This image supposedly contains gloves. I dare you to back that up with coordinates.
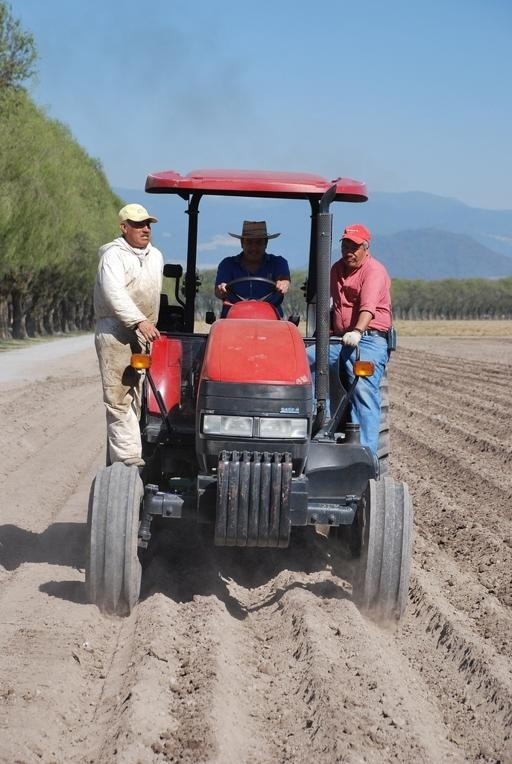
[343,331,362,348]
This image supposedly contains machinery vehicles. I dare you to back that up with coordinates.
[85,166,416,627]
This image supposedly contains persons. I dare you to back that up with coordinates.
[305,224,394,454]
[214,220,291,319]
[91,203,166,466]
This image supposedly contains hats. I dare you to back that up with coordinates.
[118,204,158,225]
[339,225,371,245]
[229,221,281,240]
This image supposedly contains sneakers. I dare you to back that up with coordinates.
[124,457,146,468]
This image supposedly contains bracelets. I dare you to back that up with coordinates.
[353,326,365,335]
